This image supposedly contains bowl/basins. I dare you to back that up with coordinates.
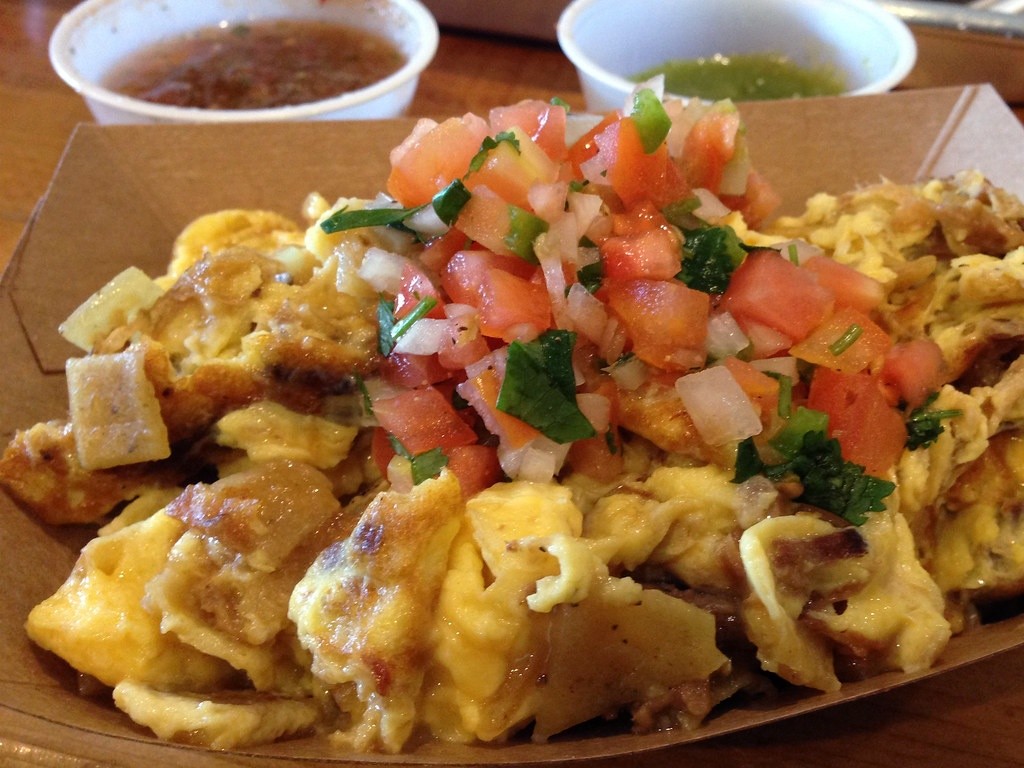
[48,0,442,120]
[556,0,918,118]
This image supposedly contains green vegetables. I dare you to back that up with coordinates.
[318,88,964,530]
